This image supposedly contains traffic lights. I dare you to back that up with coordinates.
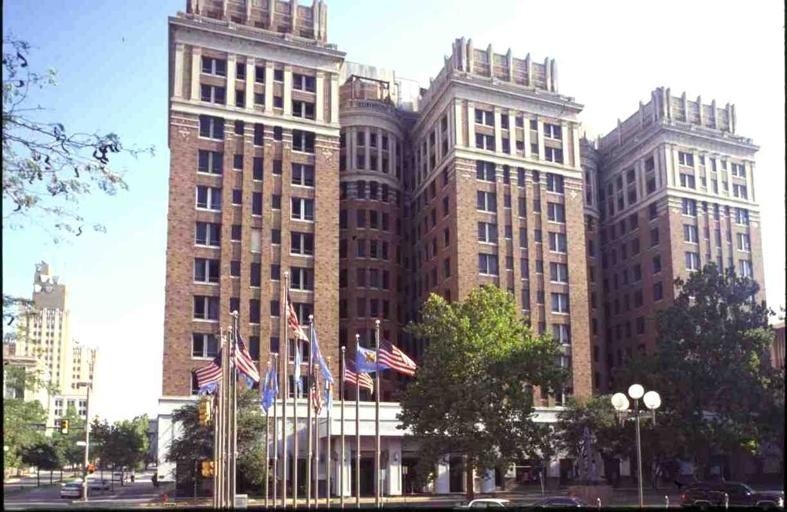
[61,419,68,435]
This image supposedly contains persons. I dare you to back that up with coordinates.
[130,468,135,484]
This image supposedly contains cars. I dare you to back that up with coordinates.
[60,470,131,498]
[454,495,591,509]
[680,481,784,509]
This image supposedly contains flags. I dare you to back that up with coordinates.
[286,289,310,342]
[356,347,390,374]
[343,358,373,394]
[192,328,336,413]
[378,335,417,376]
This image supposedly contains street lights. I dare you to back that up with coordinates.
[579,426,597,498]
[611,384,661,508]
